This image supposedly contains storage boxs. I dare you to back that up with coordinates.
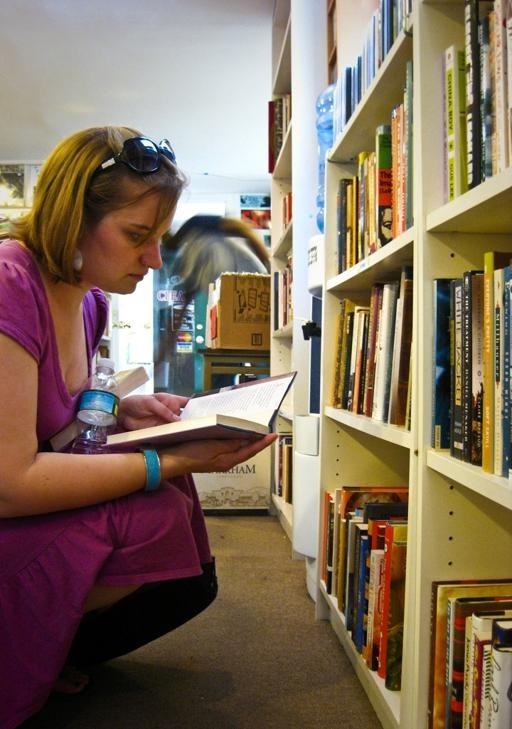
[201,271,271,353]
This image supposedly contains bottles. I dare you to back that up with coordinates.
[70,358,121,455]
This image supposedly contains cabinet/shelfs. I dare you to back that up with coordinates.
[266,0,512,728]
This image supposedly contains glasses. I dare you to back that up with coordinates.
[91,136,177,179]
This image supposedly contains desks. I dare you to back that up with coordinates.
[196,349,270,395]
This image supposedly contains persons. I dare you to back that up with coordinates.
[1,122,282,728]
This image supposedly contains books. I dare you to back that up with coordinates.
[432,252,512,479]
[333,0,413,274]
[428,578,512,729]
[267,93,293,504]
[446,0,512,202]
[101,370,299,437]
[319,265,413,691]
[47,366,150,451]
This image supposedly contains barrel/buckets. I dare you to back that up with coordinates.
[313,82,337,234]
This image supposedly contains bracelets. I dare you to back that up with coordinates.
[139,449,161,492]
